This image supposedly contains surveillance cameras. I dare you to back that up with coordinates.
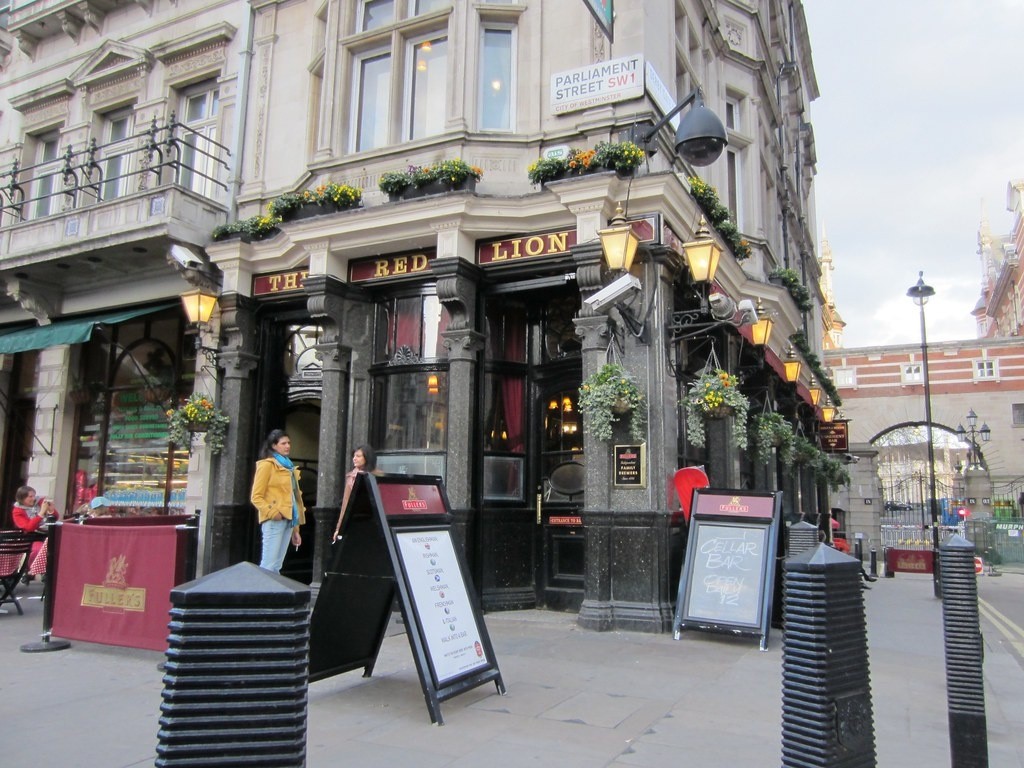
[739,299,758,325]
[583,274,642,315]
[853,456,860,462]
[171,245,203,271]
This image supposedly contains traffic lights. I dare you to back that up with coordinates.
[958,506,966,516]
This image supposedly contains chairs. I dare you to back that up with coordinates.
[1,530,48,614]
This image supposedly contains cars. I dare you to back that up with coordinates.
[884,500,913,511]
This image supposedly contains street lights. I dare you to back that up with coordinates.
[905,269,943,600]
[955,406,992,470]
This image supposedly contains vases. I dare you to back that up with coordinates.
[694,403,734,422]
[388,175,476,202]
[541,165,630,193]
[755,434,782,448]
[769,277,799,304]
[718,229,737,257]
[216,231,261,241]
[794,452,808,464]
[610,401,629,415]
[282,198,352,222]
[186,421,207,433]
[690,189,717,226]
[834,473,845,484]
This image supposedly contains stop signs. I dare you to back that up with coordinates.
[974,558,983,574]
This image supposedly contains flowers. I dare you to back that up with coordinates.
[165,392,231,457]
[577,363,647,443]
[378,157,483,194]
[676,368,751,450]
[527,140,646,190]
[688,176,751,260]
[768,263,813,312]
[748,412,851,492]
[212,180,364,238]
[791,329,842,406]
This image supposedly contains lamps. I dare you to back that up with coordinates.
[417,59,427,71]
[982,497,990,505]
[778,344,802,409]
[549,396,573,413]
[665,214,724,334]
[736,297,775,381]
[595,201,650,347]
[795,372,822,417]
[179,283,225,373]
[968,498,976,505]
[809,395,839,439]
[422,42,432,54]
[864,498,873,505]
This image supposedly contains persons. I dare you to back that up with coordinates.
[14,487,59,585]
[251,430,306,572]
[332,446,382,540]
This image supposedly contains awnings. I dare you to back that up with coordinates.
[0,303,190,453]
[743,344,787,385]
[782,379,826,423]
[688,281,753,345]
